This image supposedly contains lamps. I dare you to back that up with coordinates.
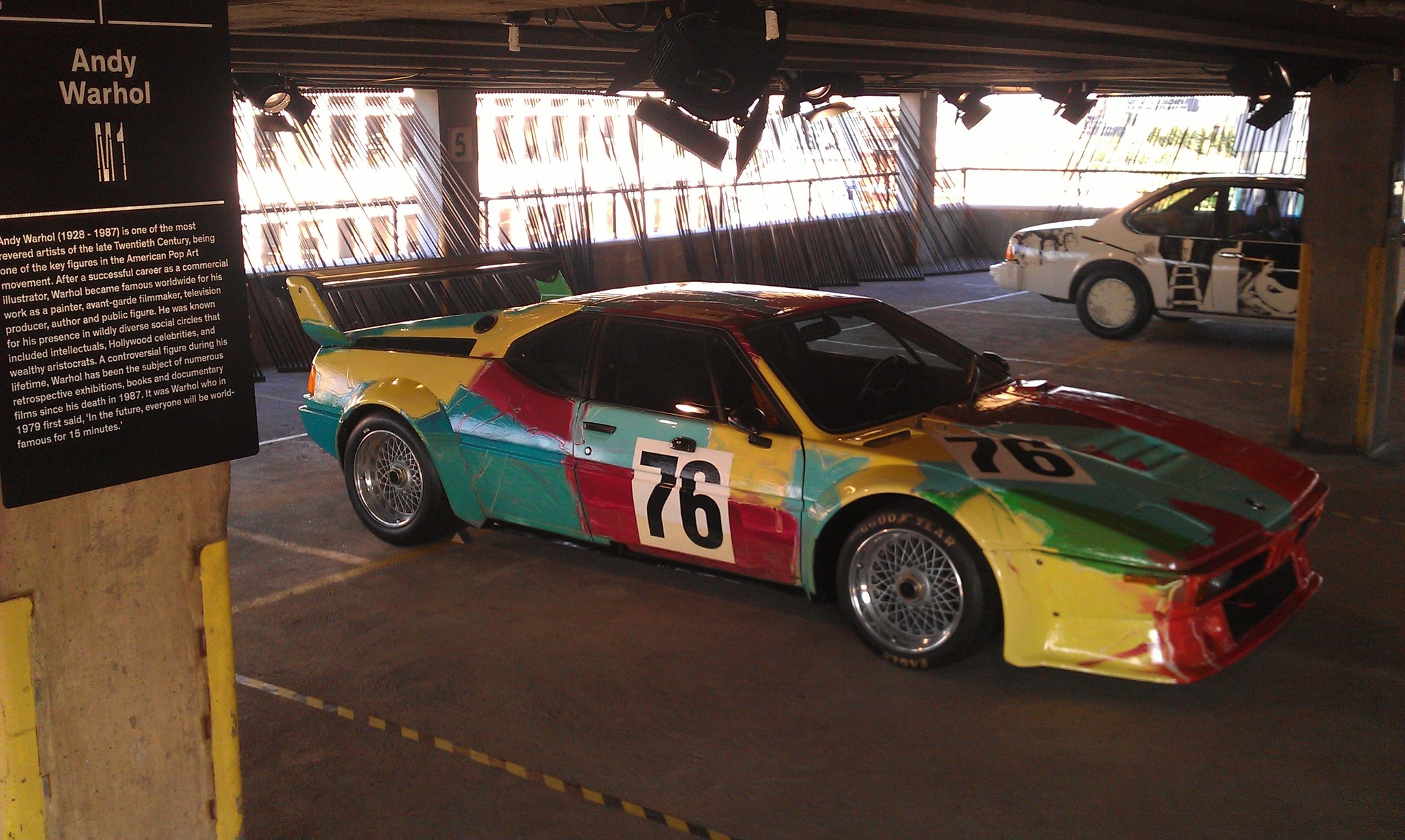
[939,87,994,128]
[1223,56,1351,131]
[606,0,864,178]
[1031,82,1097,124]
[233,72,315,133]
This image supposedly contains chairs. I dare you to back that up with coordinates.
[1160,203,1294,244]
[535,323,833,413]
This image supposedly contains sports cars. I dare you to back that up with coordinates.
[986,175,1405,342]
[284,249,1330,689]
